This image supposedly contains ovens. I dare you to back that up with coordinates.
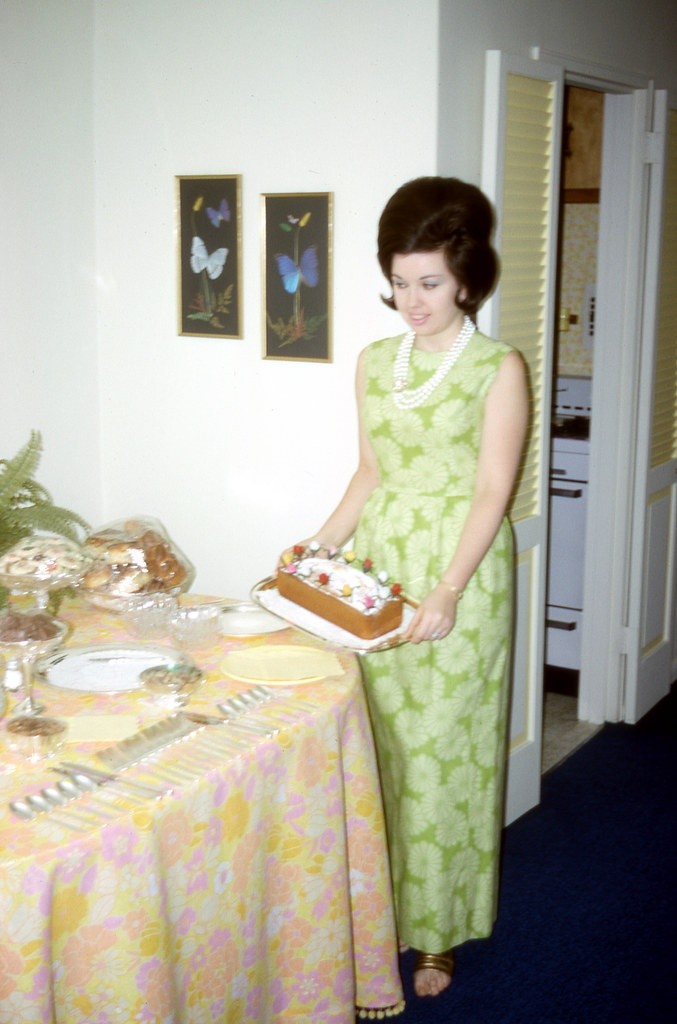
[545,451,588,691]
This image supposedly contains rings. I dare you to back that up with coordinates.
[431,632,440,639]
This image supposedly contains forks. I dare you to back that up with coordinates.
[97,715,258,787]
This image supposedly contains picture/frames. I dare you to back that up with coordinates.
[262,190,337,364]
[172,174,245,340]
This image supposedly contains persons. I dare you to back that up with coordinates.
[273,178,529,996]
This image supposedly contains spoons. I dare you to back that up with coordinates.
[42,790,116,819]
[57,781,131,814]
[237,693,303,721]
[10,801,92,836]
[72,774,147,806]
[248,689,314,716]
[257,686,321,709]
[228,699,294,727]
[26,795,106,829]
[218,704,285,732]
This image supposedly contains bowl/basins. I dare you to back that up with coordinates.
[0,621,68,650]
[5,717,68,752]
[140,663,202,694]
[75,573,195,615]
[120,593,220,652]
[0,568,89,592]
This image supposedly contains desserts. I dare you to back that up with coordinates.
[0,534,88,580]
[276,539,405,640]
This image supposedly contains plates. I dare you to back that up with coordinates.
[215,602,290,636]
[29,643,186,695]
[222,645,326,686]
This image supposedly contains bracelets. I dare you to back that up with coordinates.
[438,581,463,600]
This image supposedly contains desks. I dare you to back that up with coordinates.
[0,591,406,1024]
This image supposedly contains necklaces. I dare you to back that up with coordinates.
[391,315,477,411]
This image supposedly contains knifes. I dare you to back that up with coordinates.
[49,767,161,800]
[60,762,174,796]
[170,710,280,739]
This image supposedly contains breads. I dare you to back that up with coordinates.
[75,518,188,611]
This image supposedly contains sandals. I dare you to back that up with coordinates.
[397,937,410,954]
[413,951,456,1000]
